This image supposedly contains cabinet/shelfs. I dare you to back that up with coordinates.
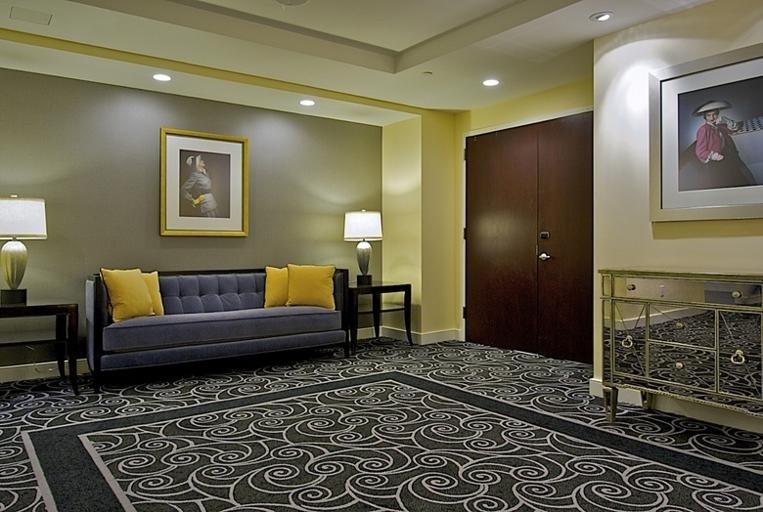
[594,270,762,426]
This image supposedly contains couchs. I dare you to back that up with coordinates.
[81,264,350,391]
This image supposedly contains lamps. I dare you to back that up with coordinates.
[0,192,49,303]
[340,211,384,284]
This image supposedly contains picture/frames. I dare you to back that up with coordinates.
[644,42,762,227]
[157,126,250,238]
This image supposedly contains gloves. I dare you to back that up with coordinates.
[716,116,729,125]
[711,152,723,161]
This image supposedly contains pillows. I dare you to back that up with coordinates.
[283,261,336,311]
[141,271,164,316]
[263,265,290,308]
[100,267,154,322]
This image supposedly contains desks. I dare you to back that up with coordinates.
[0,296,83,399]
[345,281,414,353]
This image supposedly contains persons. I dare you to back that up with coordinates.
[693,100,757,188]
[181,156,220,217]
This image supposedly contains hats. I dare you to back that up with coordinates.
[692,101,732,115]
